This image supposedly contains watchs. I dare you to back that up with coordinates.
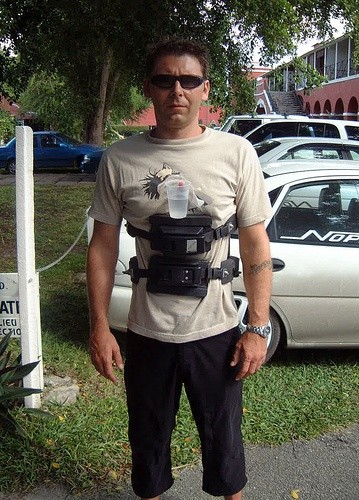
[247,324,270,339]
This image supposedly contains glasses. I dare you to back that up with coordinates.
[150,74,203,89]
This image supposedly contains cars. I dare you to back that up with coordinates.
[0,131,107,175]
[85,158,358,366]
[248,137,358,171]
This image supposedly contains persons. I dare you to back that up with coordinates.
[85,40,273,500]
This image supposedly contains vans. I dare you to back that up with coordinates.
[217,116,313,145]
[238,120,358,152]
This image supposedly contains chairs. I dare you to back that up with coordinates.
[317,187,339,218]
[348,197,359,235]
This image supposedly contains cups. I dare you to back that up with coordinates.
[166,179,191,219]
